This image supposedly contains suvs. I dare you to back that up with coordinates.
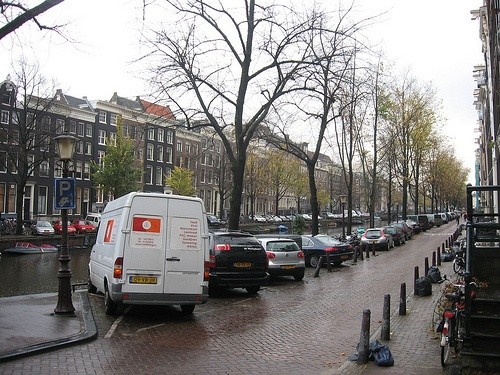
[208,229,305,296]
[207,214,266,226]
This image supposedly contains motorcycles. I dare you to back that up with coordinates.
[333,234,361,258]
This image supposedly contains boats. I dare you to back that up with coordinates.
[3,241,72,253]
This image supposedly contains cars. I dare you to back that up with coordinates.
[31,220,97,237]
[301,234,355,268]
[361,220,422,251]
[261,214,323,223]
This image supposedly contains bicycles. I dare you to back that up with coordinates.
[437,237,480,368]
[0,216,30,236]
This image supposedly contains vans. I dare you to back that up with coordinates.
[85,212,102,232]
[87,191,210,316]
[398,210,461,232]
[322,210,370,219]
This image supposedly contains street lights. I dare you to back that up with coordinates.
[395,200,400,224]
[339,193,348,238]
[419,203,422,215]
